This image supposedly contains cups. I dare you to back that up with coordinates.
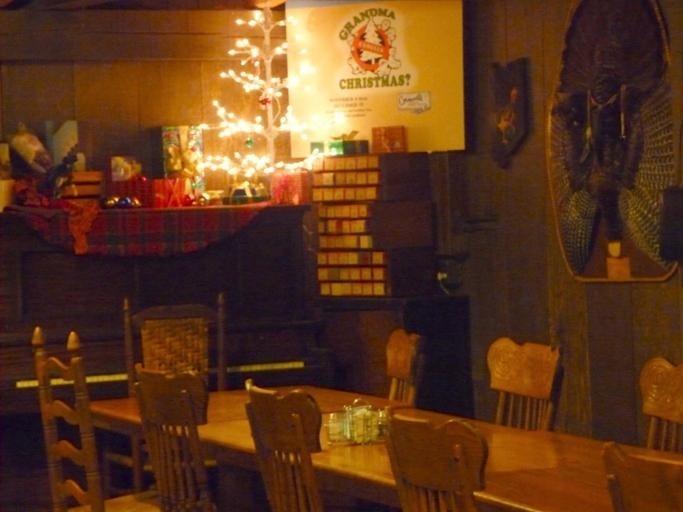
[326,398,388,446]
